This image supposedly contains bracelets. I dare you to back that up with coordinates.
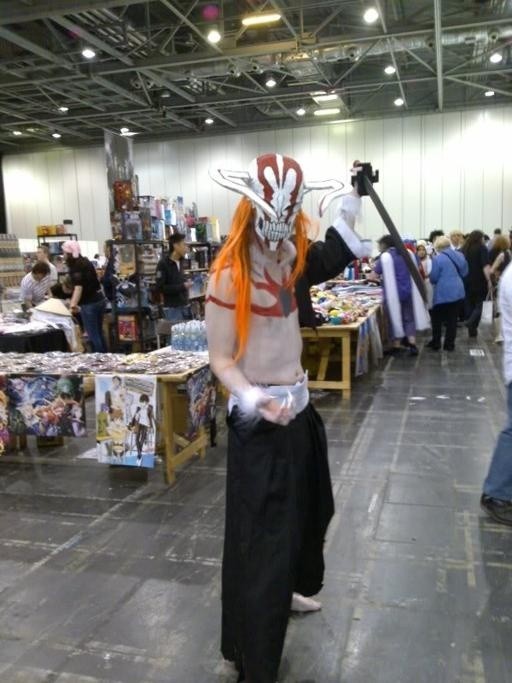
[238,387,269,417]
[341,196,362,216]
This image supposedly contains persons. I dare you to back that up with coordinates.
[480,231,512,526]
[370,228,512,357]
[96,410,131,456]
[203,153,378,683]
[19,239,117,355]
[105,376,127,424]
[155,234,194,345]
[128,395,155,467]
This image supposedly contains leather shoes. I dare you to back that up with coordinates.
[480,493,512,528]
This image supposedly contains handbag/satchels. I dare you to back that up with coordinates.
[481,289,496,325]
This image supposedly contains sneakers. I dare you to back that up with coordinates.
[291,592,321,612]
[387,340,456,358]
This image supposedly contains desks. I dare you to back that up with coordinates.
[298,254,389,399]
[0,227,229,486]
